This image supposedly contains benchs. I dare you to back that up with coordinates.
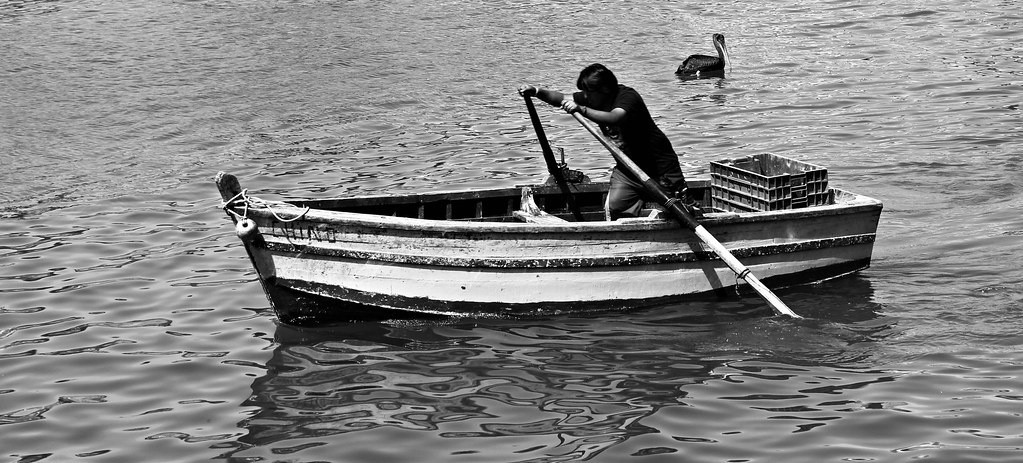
[516,208,569,227]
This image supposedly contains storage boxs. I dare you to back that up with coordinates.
[708,151,830,214]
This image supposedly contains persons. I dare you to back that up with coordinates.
[519,63,694,222]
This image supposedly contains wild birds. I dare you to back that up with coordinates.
[674,33,732,74]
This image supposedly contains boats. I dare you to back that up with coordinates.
[214,171,885,333]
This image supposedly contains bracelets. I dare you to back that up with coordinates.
[533,86,539,96]
[583,105,587,114]
